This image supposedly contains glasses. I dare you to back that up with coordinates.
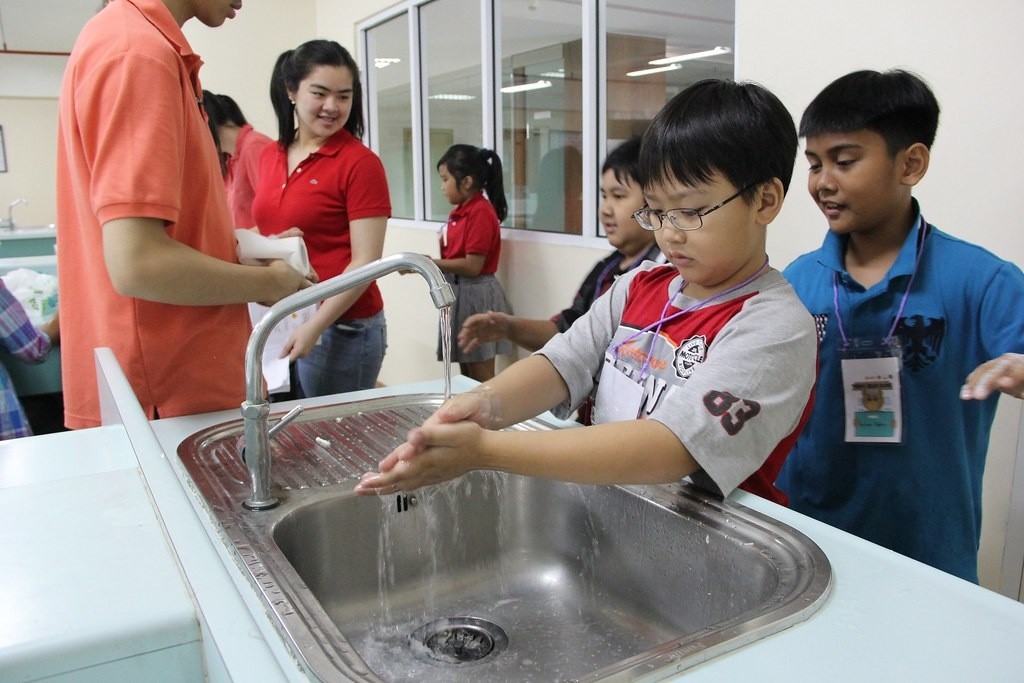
[631,183,752,232]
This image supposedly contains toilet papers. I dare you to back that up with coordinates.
[234,225,310,280]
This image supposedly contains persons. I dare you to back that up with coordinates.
[353,78,821,498]
[458,137,668,354]
[252,39,393,398]
[397,144,512,383]
[58,1,319,429]
[201,89,275,230]
[780,71,1024,588]
[0,278,60,441]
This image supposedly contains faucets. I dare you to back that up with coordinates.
[241,255,455,510]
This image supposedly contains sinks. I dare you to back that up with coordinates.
[176,393,832,682]
